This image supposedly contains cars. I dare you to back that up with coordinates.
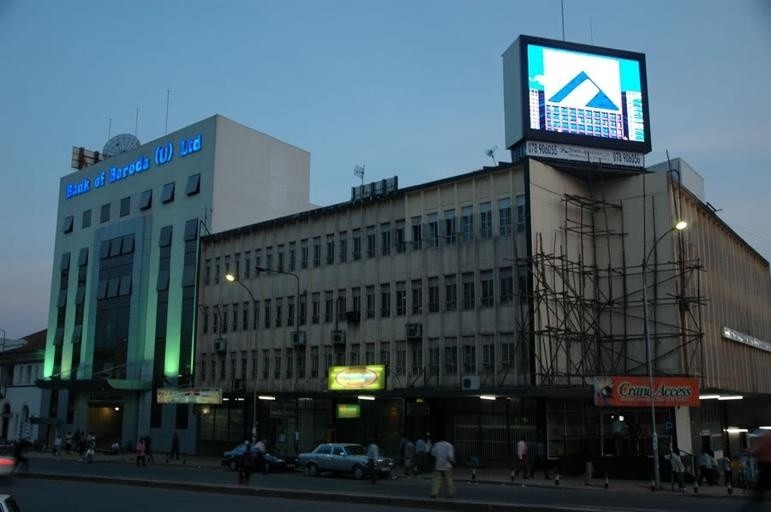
[0,495,20,512]
[221,443,393,480]
[0,455,16,476]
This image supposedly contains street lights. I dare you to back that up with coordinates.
[226,265,300,450]
[643,220,689,491]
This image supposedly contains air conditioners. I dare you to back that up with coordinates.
[291,331,307,346]
[331,331,345,344]
[214,339,226,351]
[406,324,421,338]
[462,375,480,391]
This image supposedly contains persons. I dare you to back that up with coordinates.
[136,436,153,467]
[510,433,553,481]
[168,431,179,461]
[367,438,379,484]
[671,448,750,495]
[401,434,432,477]
[52,429,96,456]
[430,432,456,498]
[232,437,269,485]
[111,439,119,454]
